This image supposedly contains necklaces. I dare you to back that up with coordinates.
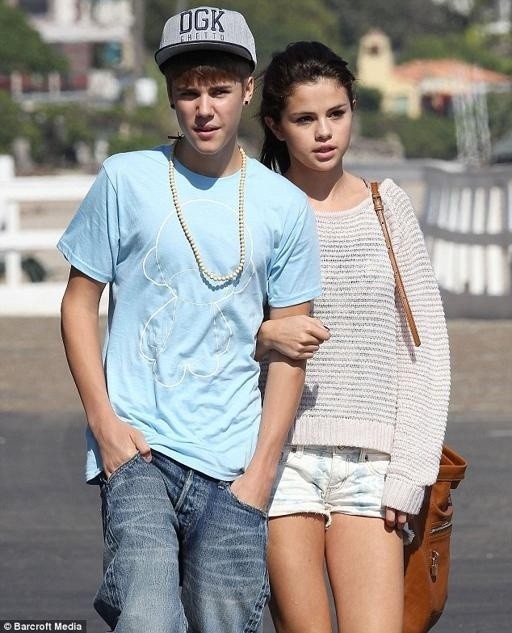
[166,135,248,281]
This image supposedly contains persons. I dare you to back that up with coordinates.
[253,38,453,633]
[55,4,323,633]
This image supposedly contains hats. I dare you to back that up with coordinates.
[153,7,259,78]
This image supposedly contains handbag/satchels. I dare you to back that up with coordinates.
[366,174,468,632]
